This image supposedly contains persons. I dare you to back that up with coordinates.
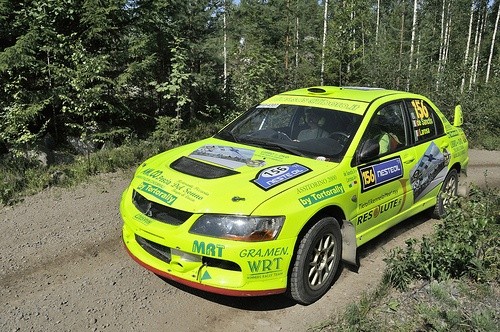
[352,114,391,157]
[296,106,329,142]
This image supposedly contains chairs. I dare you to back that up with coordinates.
[377,114,404,148]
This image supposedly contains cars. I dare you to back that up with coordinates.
[117,84,469,306]
